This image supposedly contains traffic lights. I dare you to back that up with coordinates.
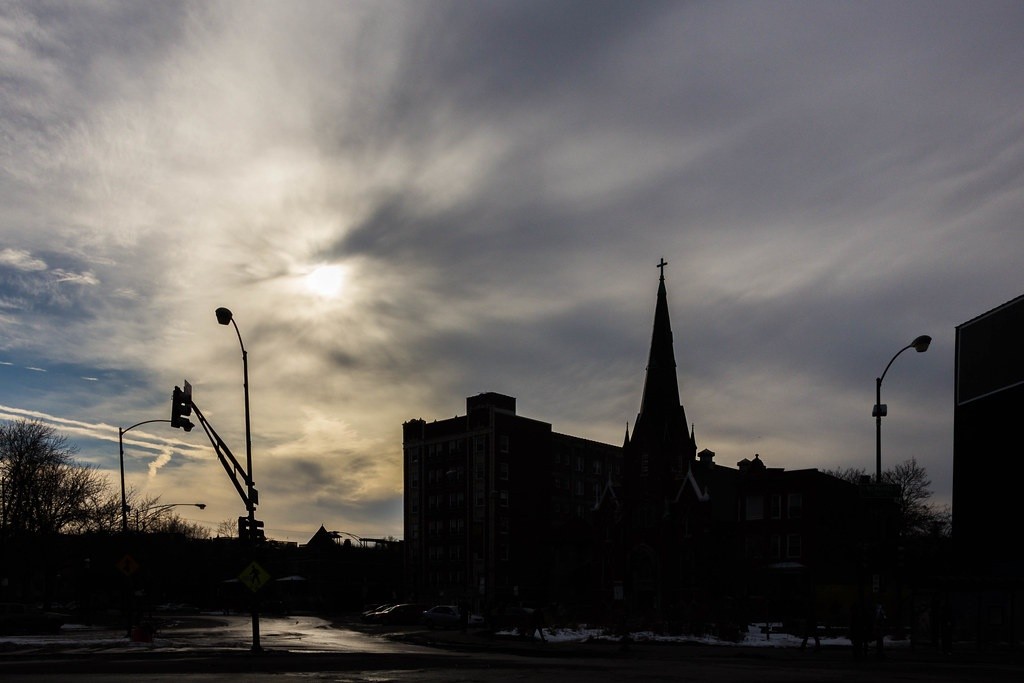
[171,387,194,433]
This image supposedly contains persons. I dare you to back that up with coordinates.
[529,610,546,645]
[800,592,821,651]
[460,607,472,633]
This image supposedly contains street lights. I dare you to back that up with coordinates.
[869,333,932,648]
[136,502,206,530]
[214,306,265,655]
